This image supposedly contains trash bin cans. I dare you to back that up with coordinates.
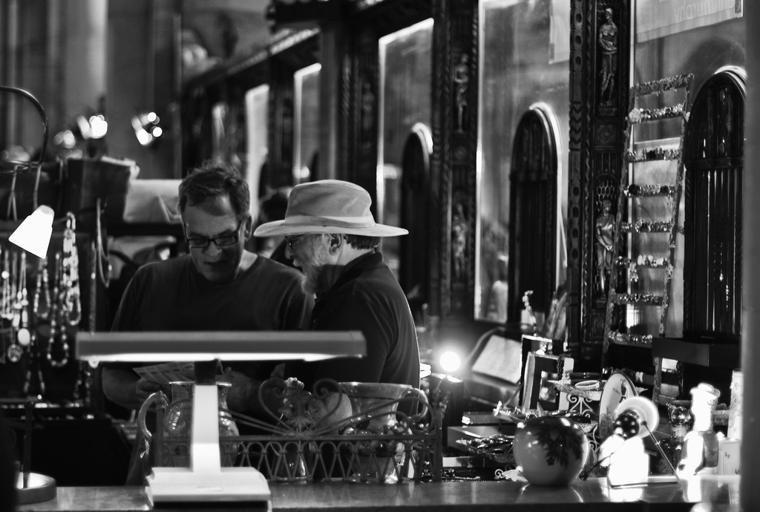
[427,316,441,337]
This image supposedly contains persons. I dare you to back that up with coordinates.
[236,181,420,483]
[102,169,314,482]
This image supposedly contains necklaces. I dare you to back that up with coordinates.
[0,212,82,401]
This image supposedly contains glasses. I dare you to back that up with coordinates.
[284,235,307,250]
[185,221,241,248]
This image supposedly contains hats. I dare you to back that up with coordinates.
[252,178,408,239]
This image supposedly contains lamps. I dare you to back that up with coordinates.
[72,327,374,510]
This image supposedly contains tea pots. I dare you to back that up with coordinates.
[137,381,240,470]
[334,381,428,481]
